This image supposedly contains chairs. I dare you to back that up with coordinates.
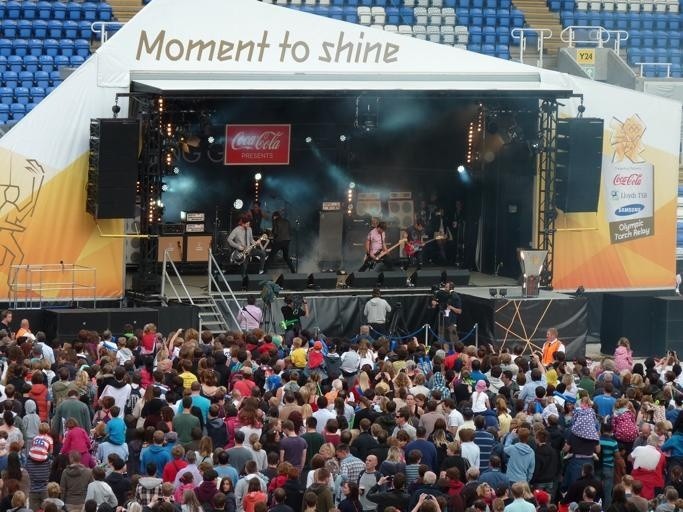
[0,0,123,123]
[261,0,683,78]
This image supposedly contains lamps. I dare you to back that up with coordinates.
[156,95,214,208]
[485,109,545,155]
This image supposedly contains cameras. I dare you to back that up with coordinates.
[157,498,163,501]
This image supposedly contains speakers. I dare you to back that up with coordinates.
[275,273,304,291]
[345,272,378,288]
[441,269,471,286]
[555,116,604,214]
[243,274,274,290]
[379,271,408,287]
[602,294,654,357]
[306,273,337,288]
[409,270,441,286]
[84,119,138,219]
[212,274,242,290]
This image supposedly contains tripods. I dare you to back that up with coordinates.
[387,310,409,335]
[261,303,276,333]
[428,312,453,345]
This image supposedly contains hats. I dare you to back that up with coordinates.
[244,367,252,374]
[314,341,322,349]
[329,344,336,349]
[34,344,43,354]
[475,380,487,392]
[533,490,549,504]
[374,382,390,395]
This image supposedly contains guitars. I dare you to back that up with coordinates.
[405,235,449,256]
[365,238,407,270]
[230,233,268,266]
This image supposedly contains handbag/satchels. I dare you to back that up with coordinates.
[55,453,70,464]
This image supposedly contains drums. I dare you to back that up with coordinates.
[265,227,273,240]
[219,230,232,250]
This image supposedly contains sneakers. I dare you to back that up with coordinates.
[594,453,599,461]
[563,453,573,459]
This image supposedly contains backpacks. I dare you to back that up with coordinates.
[126,386,142,415]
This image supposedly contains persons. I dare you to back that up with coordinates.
[363,288,392,338]
[280,296,309,346]
[225,196,467,277]
[0,308,682,511]
[431,281,463,342]
[237,294,263,331]
